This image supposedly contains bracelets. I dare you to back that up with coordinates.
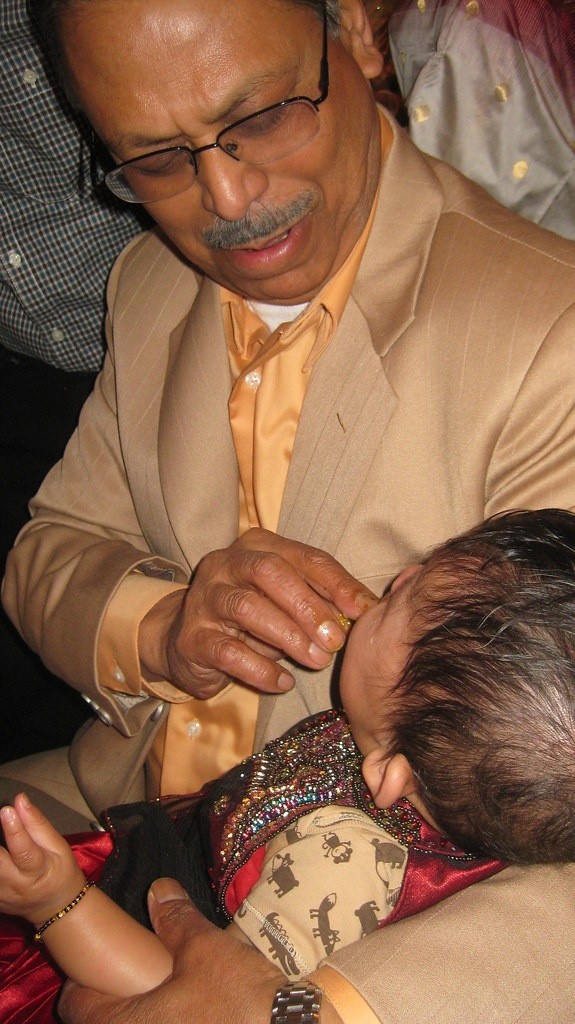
[270,980,324,1024]
[33,882,95,940]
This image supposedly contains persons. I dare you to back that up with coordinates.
[0,509,575,1024]
[0,1,574,1024]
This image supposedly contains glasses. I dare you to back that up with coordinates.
[92,0,329,205]
